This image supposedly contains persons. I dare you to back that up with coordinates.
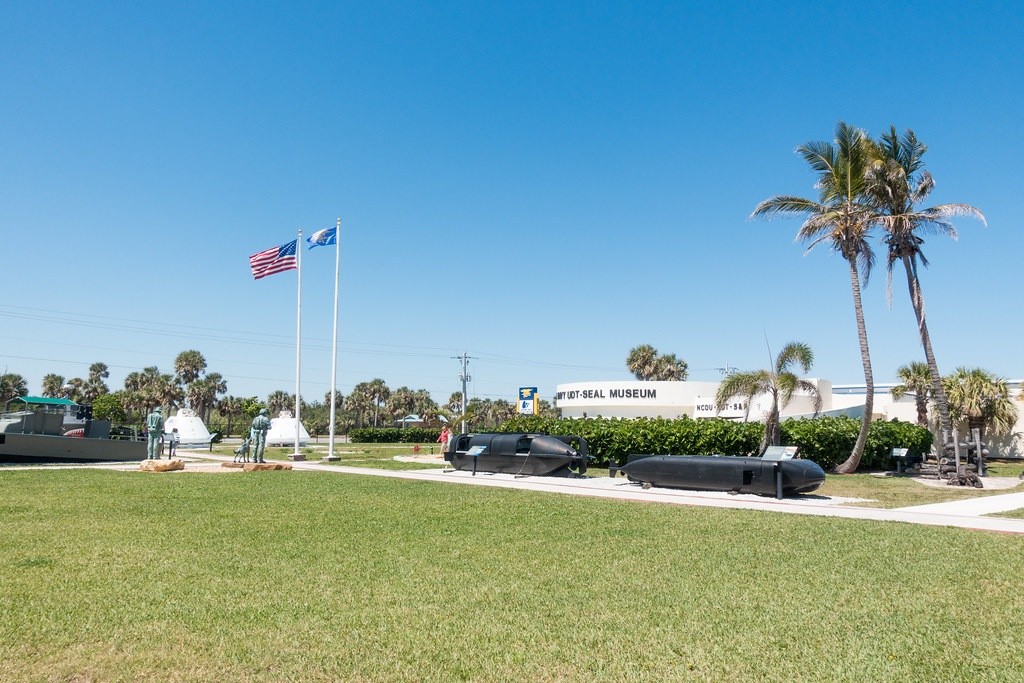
[140,424,148,441]
[146,407,163,459]
[252,409,271,463]
[437,427,448,458]
[171,428,180,456]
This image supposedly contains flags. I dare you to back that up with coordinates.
[248,239,298,280]
[307,226,337,251]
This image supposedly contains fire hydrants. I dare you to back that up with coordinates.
[430,446,433,455]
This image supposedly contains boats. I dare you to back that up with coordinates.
[0,396,164,460]
